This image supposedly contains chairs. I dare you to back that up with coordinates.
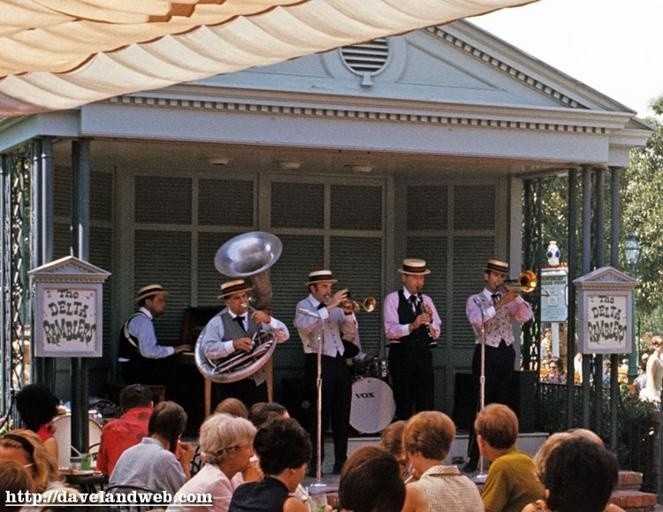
[103,484,169,512]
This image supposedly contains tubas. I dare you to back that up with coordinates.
[195,232,281,383]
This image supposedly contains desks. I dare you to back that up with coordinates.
[172,356,273,419]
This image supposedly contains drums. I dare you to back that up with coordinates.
[364,359,389,378]
[349,377,396,435]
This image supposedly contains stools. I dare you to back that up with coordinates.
[107,382,166,405]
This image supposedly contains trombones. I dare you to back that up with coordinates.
[496,271,550,297]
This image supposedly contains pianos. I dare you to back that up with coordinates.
[176,348,198,367]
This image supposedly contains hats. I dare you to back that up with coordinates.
[134,285,169,305]
[482,259,511,276]
[397,258,431,276]
[304,270,339,288]
[217,279,253,299]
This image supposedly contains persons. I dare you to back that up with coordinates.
[0,384,625,511]
[10,341,33,428]
[462,258,534,473]
[294,270,362,478]
[382,257,443,420]
[194,280,291,410]
[518,321,663,411]
[119,284,199,438]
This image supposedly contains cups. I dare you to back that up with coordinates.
[81,457,91,470]
[401,463,416,483]
[309,483,327,512]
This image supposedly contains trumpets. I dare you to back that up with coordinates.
[327,289,378,316]
